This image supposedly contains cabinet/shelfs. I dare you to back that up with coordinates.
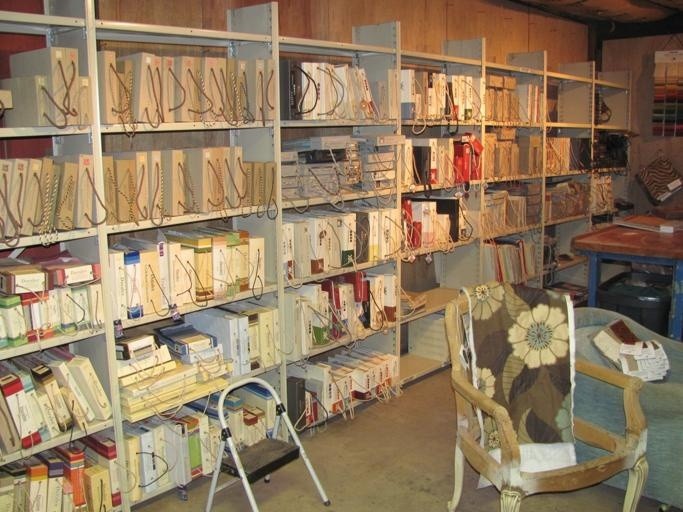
[0,1,640,511]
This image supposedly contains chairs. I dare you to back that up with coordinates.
[573,307,683,511]
[444,284,649,511]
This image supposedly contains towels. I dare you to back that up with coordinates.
[459,286,577,489]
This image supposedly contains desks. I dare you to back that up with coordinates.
[570,215,682,340]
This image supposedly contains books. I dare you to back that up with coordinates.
[483,233,536,287]
[546,280,588,299]
[614,212,672,234]
[398,288,426,321]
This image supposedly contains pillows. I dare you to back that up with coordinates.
[575,317,641,373]
